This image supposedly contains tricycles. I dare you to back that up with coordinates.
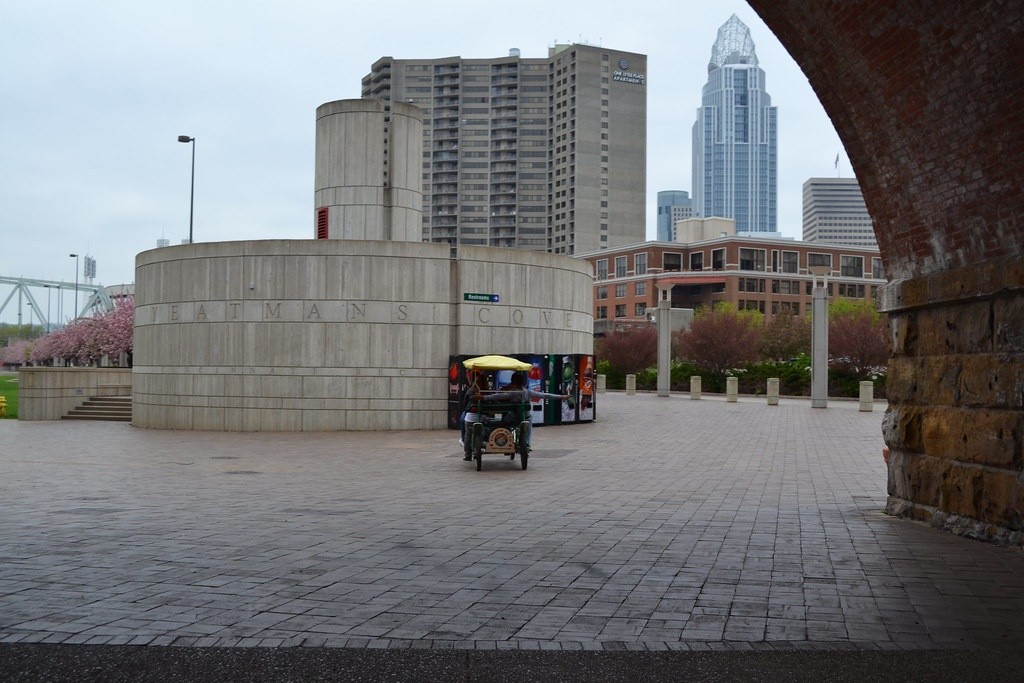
[459,355,533,472]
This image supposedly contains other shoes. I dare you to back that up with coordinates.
[459,438,465,447]
[527,446,533,451]
[463,457,472,461]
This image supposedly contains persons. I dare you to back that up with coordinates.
[459,371,571,461]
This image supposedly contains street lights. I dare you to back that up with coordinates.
[26,302,33,339]
[55,285,60,329]
[18,314,23,327]
[44,285,51,334]
[69,254,79,326]
[177,135,195,244]
